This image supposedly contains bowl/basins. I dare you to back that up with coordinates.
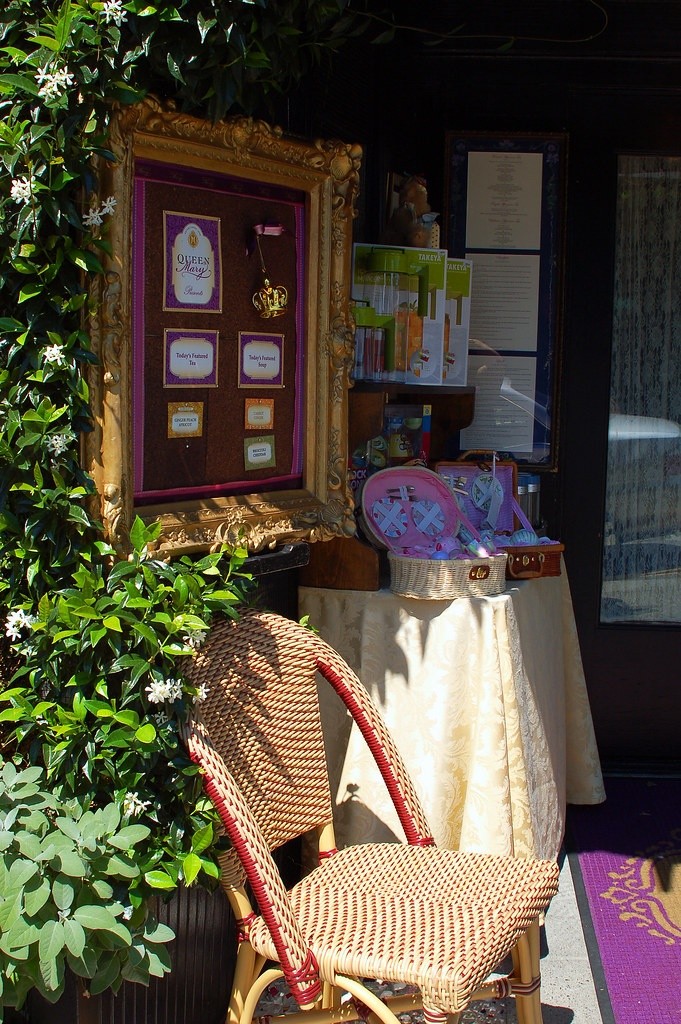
[384,416,404,429]
[404,417,422,430]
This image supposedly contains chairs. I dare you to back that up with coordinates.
[177,607,561,1024]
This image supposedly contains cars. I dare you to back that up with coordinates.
[495,377,680,568]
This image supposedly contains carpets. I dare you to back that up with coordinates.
[562,776,681,1024]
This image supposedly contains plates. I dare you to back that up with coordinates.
[371,434,390,450]
[370,451,387,468]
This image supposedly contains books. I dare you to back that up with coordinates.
[350,242,472,387]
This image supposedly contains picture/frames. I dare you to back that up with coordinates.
[75,90,359,586]
[441,125,572,476]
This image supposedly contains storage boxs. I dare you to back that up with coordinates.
[351,242,448,387]
[443,257,474,387]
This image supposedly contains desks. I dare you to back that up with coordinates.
[297,552,606,927]
